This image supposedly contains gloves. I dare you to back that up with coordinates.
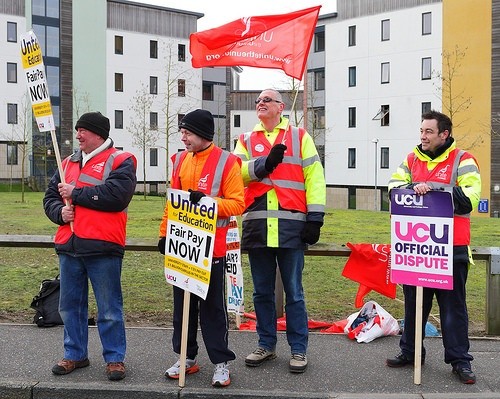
[158,238,166,256]
[188,188,206,205]
[268,144,287,164]
[301,220,322,244]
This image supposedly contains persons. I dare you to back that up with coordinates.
[387,112,479,385]
[158,110,245,387]
[233,86,329,373]
[43,111,137,381]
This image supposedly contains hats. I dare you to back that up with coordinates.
[75,112,110,140]
[179,109,214,141]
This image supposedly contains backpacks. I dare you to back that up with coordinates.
[30,274,64,328]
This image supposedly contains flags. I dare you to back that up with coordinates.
[187,4,322,82]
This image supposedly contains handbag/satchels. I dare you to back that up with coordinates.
[344,301,399,343]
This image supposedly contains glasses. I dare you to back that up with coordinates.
[255,96,282,103]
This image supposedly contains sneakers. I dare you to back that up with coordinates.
[452,365,476,384]
[52,355,89,375]
[245,344,276,367]
[106,361,126,379]
[165,356,199,379]
[289,352,307,373]
[386,353,425,367]
[211,363,230,387]
